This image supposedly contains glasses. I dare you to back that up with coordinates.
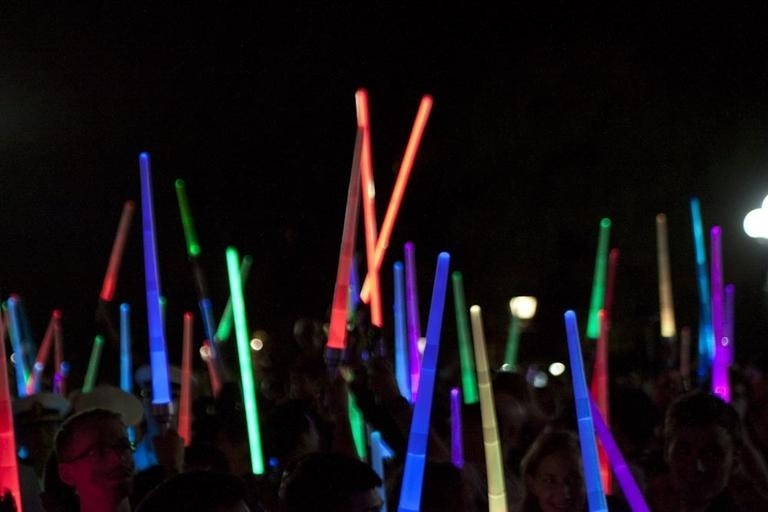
[66,437,135,462]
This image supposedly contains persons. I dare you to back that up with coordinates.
[4,311,767,512]
